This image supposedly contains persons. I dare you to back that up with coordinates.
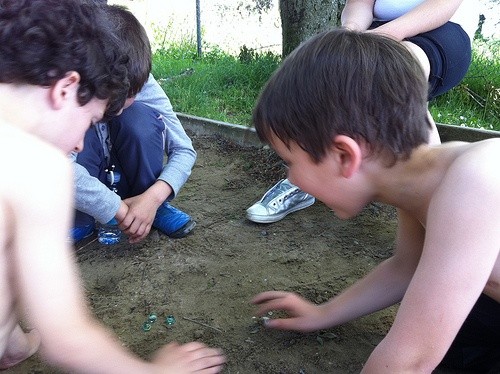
[245,0,481,224]
[251,28,500,374]
[0,0,228,374]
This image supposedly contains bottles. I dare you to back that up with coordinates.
[98,173,123,246]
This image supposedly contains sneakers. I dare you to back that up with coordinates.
[245,178,315,223]
[71,220,97,243]
[152,203,196,238]
